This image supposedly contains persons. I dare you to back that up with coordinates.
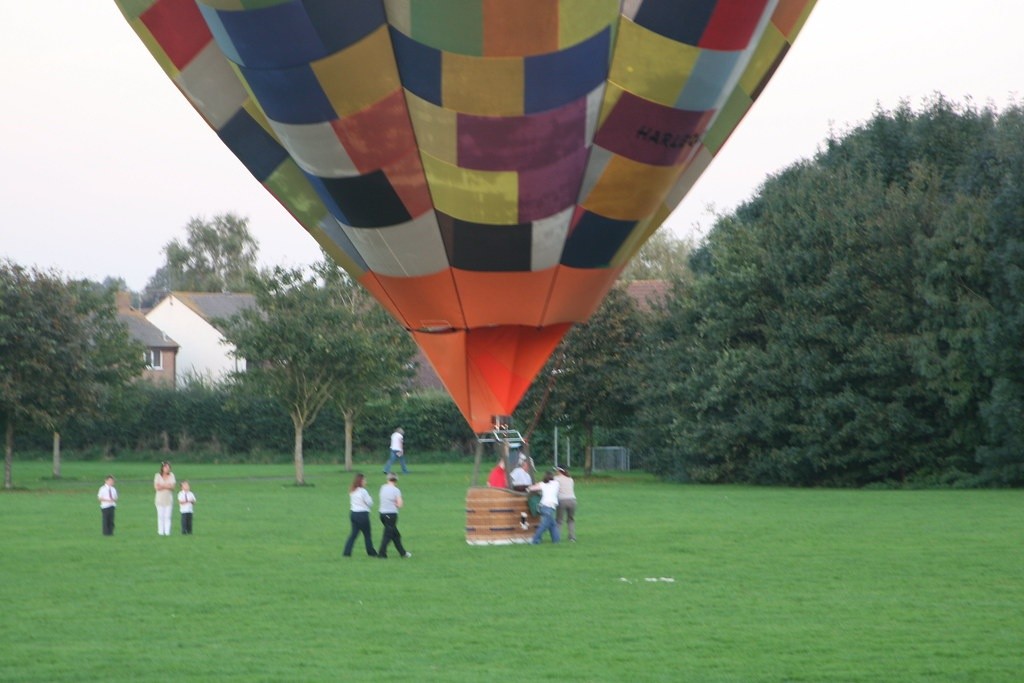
[486,457,511,489]
[382,427,410,475]
[525,472,561,544]
[551,463,576,543]
[177,480,196,535]
[343,473,378,557]
[377,472,412,558]
[97,475,119,536]
[508,460,533,530]
[154,461,177,535]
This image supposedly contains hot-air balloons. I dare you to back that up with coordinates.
[115,0,821,544]
